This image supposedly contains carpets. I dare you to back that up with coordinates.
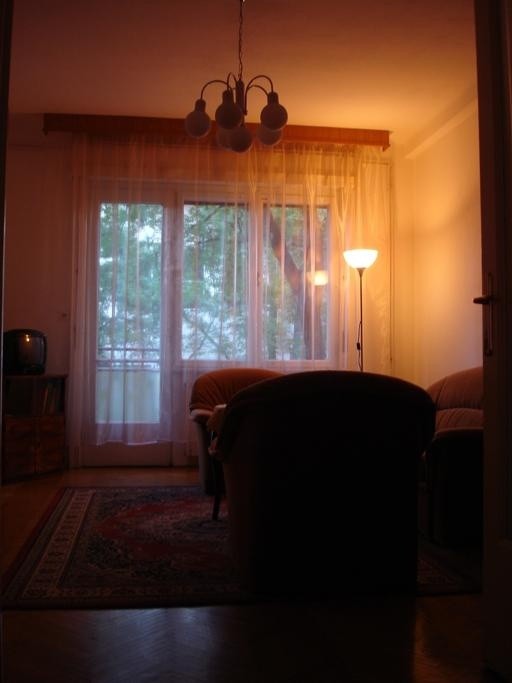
[4,485,485,608]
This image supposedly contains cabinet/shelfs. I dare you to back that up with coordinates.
[3,374,69,480]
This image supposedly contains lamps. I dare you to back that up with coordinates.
[184,0,290,154]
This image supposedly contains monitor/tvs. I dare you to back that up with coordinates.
[3,328,47,375]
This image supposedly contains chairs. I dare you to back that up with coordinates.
[205,371,436,602]
[191,369,294,522]
[416,366,482,557]
